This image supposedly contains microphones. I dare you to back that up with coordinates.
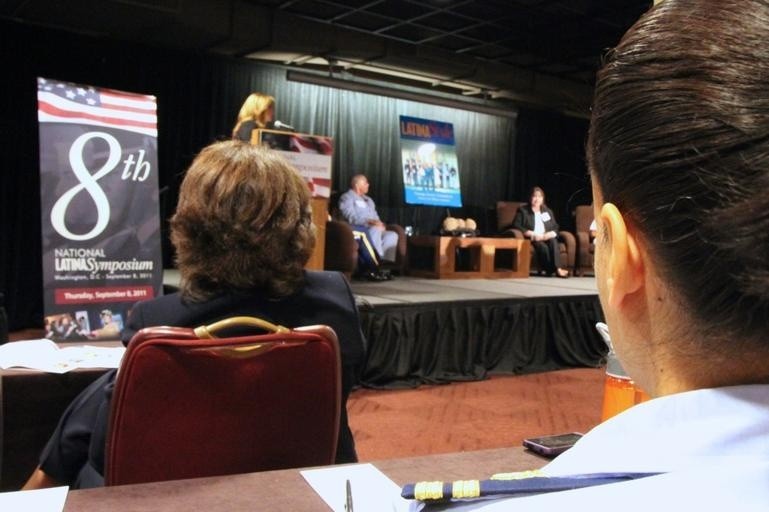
[275,120,296,131]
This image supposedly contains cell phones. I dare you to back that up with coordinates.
[521,431,586,457]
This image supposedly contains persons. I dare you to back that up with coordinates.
[230,93,277,141]
[511,186,570,278]
[9,137,373,488]
[391,0,769,512]
[98,308,114,327]
[338,173,400,282]
[400,158,455,196]
[42,311,87,341]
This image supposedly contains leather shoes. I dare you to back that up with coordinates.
[369,272,390,281]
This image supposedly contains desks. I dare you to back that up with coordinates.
[0,446,554,512]
[0,339,126,492]
[405,234,531,279]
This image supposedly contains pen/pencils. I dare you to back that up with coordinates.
[345,480,352,512]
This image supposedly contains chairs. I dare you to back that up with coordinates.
[103,317,342,488]
[495,202,576,277]
[325,190,408,282]
[573,205,595,277]
